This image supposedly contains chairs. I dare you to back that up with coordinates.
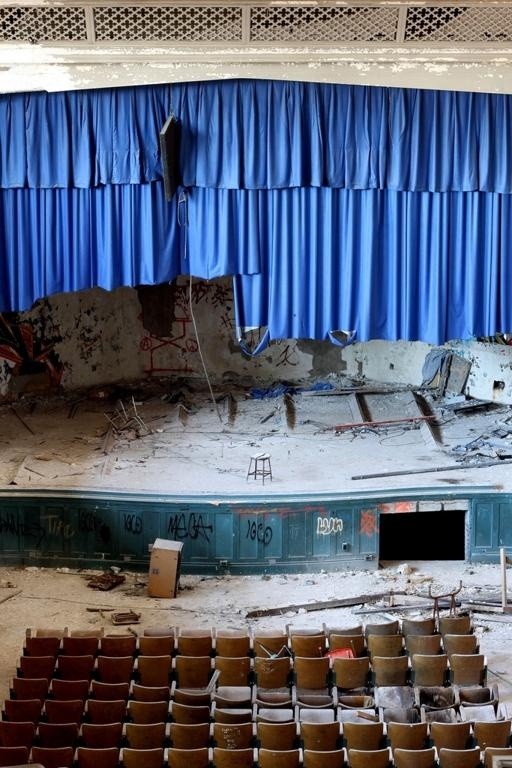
[1,607,512,768]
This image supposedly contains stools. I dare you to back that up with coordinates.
[246,452,274,485]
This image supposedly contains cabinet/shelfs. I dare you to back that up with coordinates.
[145,537,185,598]
